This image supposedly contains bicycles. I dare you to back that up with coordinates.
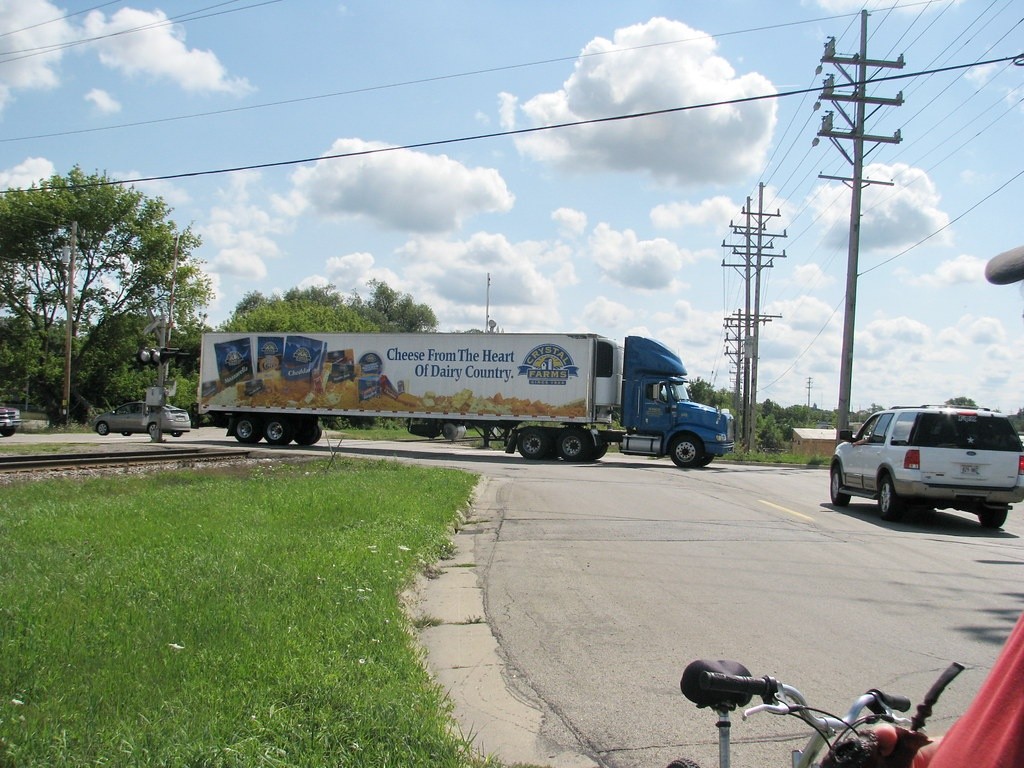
[667,657,912,768]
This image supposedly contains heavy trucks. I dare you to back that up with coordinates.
[197,332,735,468]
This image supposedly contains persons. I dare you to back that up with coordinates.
[873,610,1024,768]
[852,439,869,448]
[659,384,667,402]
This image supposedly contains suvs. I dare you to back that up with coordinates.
[829,405,1024,528]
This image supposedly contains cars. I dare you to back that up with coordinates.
[0,403,21,436]
[92,401,192,437]
